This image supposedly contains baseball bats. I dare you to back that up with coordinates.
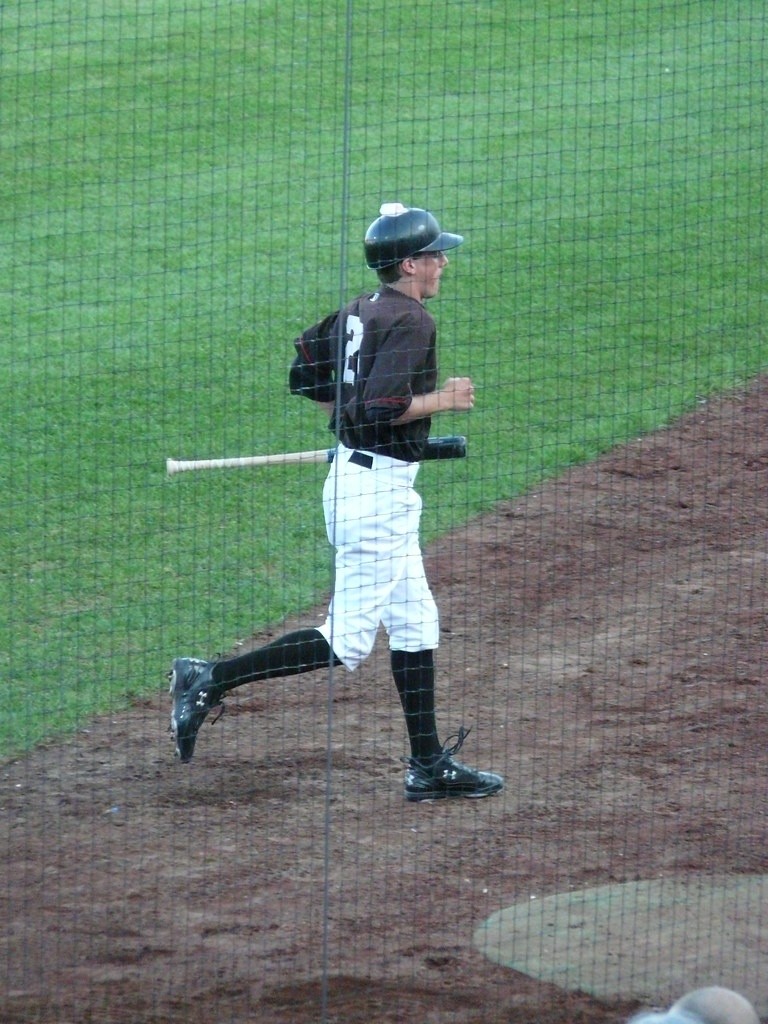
[166,433,470,477]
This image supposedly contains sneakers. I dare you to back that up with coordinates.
[400,726,504,801]
[168,657,227,764]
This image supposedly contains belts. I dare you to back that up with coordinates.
[348,451,373,469]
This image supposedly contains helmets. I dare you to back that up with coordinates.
[365,208,464,269]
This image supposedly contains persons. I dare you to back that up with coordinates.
[167,202,505,803]
